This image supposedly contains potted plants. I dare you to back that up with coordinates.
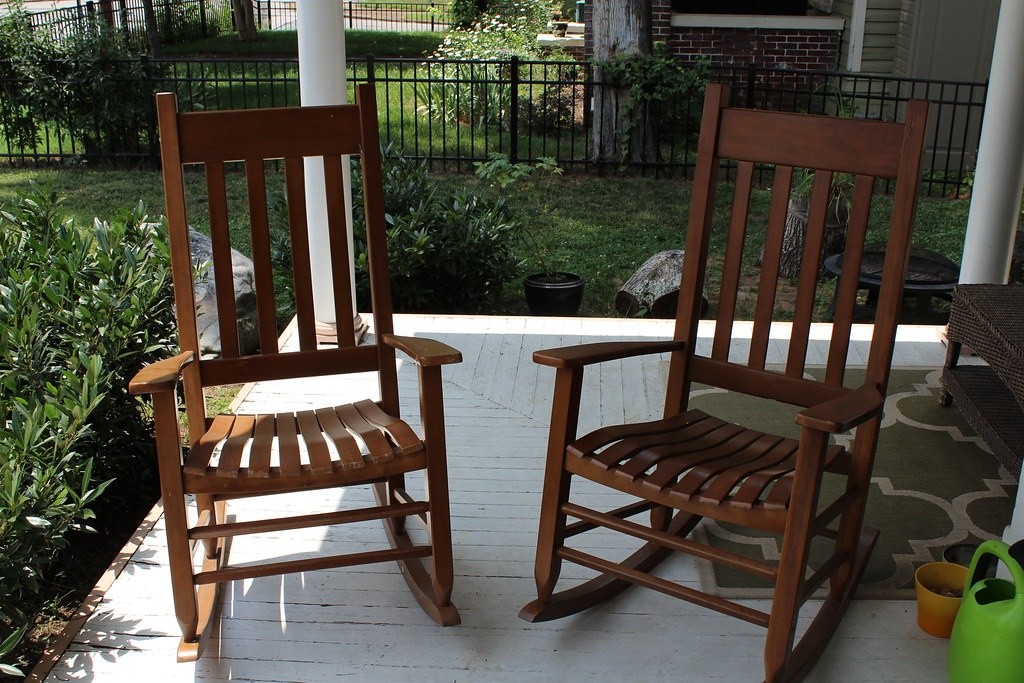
[472,152,586,316]
[791,70,860,225]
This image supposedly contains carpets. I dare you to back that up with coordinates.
[659,362,1022,600]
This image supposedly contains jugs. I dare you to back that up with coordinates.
[947,540,1024,683]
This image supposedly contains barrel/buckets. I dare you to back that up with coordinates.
[914,562,969,638]
[942,543,980,568]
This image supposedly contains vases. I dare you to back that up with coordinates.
[916,561,968,640]
[942,544,999,579]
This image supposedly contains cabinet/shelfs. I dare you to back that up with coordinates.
[937,282,1024,485]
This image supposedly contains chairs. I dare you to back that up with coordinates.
[128,84,464,665]
[517,83,930,683]
